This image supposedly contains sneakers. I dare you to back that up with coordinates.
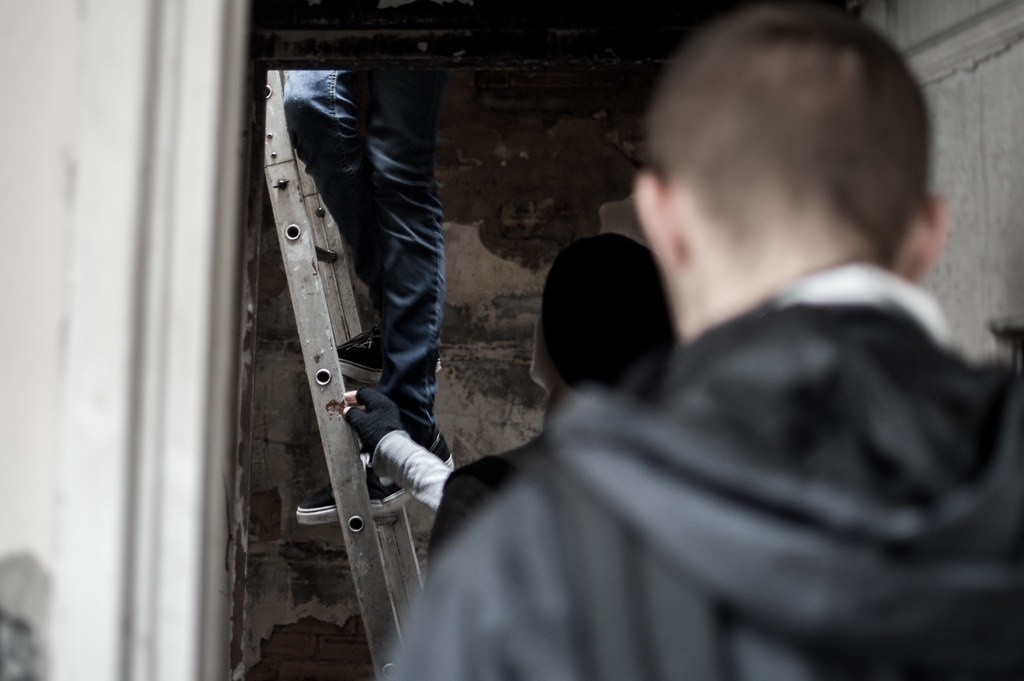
[335,328,445,386]
[297,436,456,527]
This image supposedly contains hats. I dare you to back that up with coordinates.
[540,236,677,391]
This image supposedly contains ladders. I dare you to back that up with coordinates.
[260,71,424,680]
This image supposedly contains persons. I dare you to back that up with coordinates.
[371,5,1024,681]
[279,63,459,526]
[340,230,680,584]
